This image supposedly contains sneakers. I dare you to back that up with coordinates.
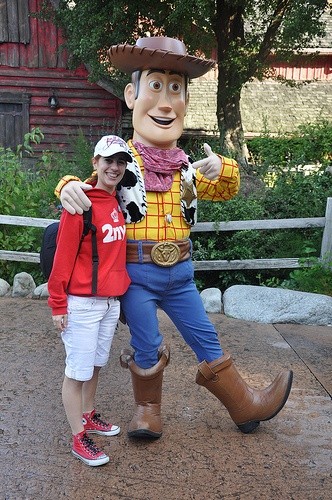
[81,409,120,436]
[72,430,109,467]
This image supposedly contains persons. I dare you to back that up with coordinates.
[48,135,131,467]
[54,36,293,436]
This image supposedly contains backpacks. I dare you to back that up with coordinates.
[40,194,122,280]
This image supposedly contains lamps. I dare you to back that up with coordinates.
[48,89,58,109]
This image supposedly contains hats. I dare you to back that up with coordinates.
[94,134,133,163]
